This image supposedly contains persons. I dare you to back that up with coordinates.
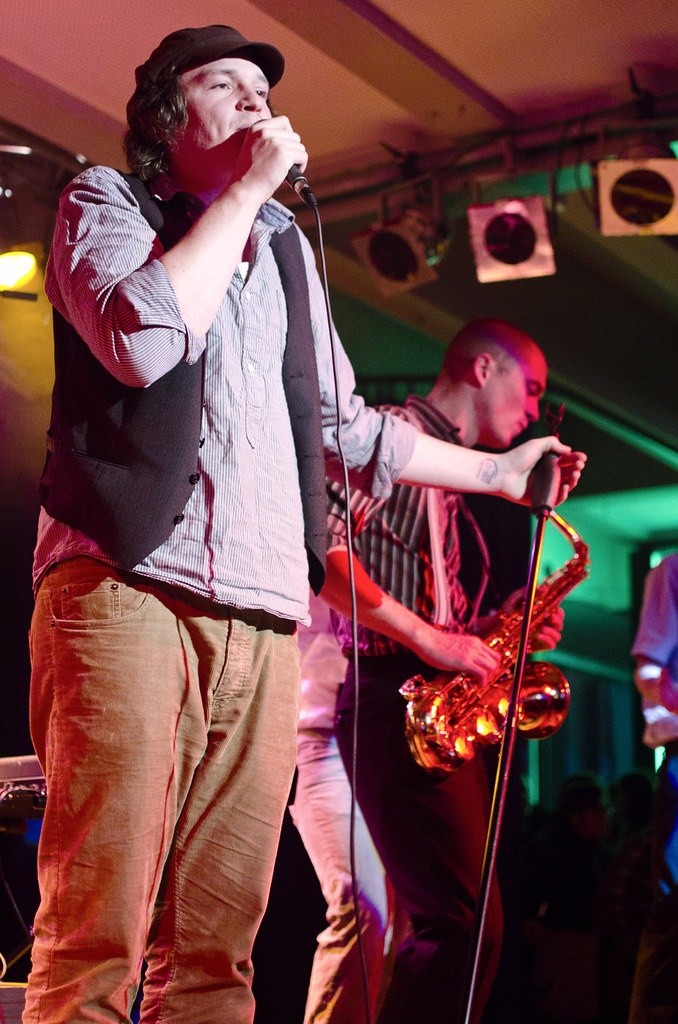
[25,24,590,1024]
[282,317,550,1023]
[501,769,663,1024]
[630,545,678,911]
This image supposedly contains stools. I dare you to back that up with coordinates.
[0,754,48,982]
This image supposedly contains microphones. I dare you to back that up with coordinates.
[286,165,320,212]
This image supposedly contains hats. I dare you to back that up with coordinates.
[125,24,286,127]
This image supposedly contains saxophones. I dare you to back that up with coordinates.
[400,509,593,774]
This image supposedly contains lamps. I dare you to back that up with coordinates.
[0,175,38,294]
[350,190,464,300]
[467,193,563,286]
[598,139,678,238]
